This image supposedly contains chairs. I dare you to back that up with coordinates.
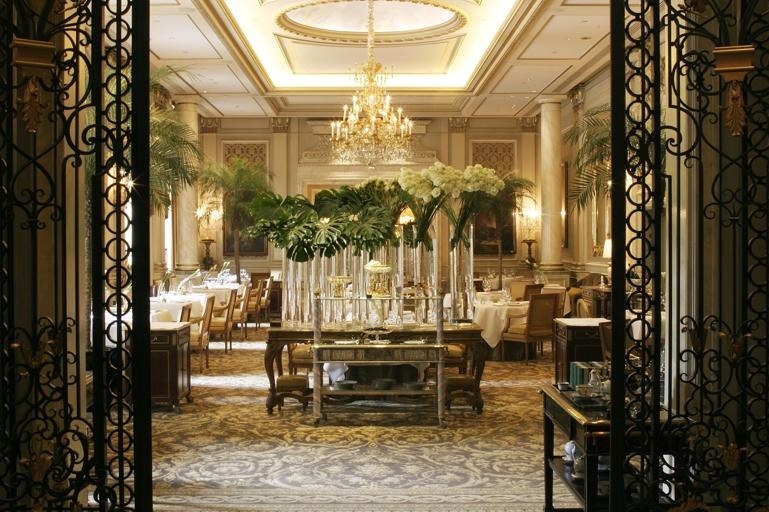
[149,276,274,373]
[598,317,636,362]
[476,274,591,365]
[423,342,468,381]
[286,342,313,375]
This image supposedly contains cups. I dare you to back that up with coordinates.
[575,384,592,397]
[557,382,569,390]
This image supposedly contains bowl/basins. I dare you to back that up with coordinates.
[337,381,358,390]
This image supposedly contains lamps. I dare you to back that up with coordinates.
[327,2,417,165]
[520,191,539,271]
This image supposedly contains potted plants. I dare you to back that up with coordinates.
[236,177,412,326]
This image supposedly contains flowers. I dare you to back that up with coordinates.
[399,161,506,251]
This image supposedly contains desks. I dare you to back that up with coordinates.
[536,382,701,512]
[264,322,486,415]
[591,284,611,317]
[122,321,194,414]
[552,316,612,383]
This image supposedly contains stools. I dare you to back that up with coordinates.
[276,374,307,411]
[445,374,475,410]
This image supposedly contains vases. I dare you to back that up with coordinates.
[414,239,474,326]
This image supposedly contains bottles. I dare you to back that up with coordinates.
[588,369,602,397]
